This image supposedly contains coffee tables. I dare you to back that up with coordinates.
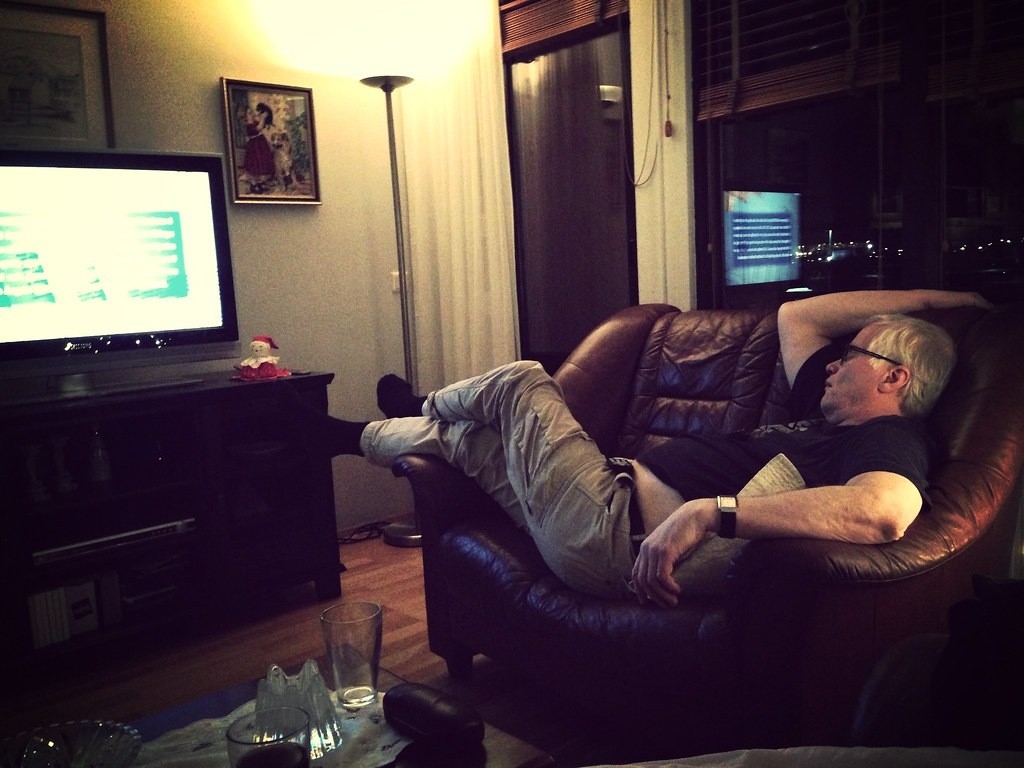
[63,644,558,768]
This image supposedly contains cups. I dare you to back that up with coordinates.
[320,600,383,708]
[226,706,311,768]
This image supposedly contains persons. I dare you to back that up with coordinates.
[282,289,995,606]
[238,105,277,194]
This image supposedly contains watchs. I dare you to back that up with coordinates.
[716,494,739,539]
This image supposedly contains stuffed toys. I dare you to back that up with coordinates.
[239,336,291,380]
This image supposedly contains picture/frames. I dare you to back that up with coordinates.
[1,2,115,150]
[220,76,324,206]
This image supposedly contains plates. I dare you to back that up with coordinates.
[0,719,142,768]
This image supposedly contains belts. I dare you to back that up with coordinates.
[607,456,646,558]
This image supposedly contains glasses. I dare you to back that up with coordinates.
[839,341,903,372]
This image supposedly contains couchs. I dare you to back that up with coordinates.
[392,304,1024,749]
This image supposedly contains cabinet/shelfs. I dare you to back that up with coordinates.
[0,366,341,702]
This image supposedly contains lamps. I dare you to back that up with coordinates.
[363,75,425,548]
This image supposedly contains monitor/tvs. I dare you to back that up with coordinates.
[0,146,242,407]
[725,184,807,295]
